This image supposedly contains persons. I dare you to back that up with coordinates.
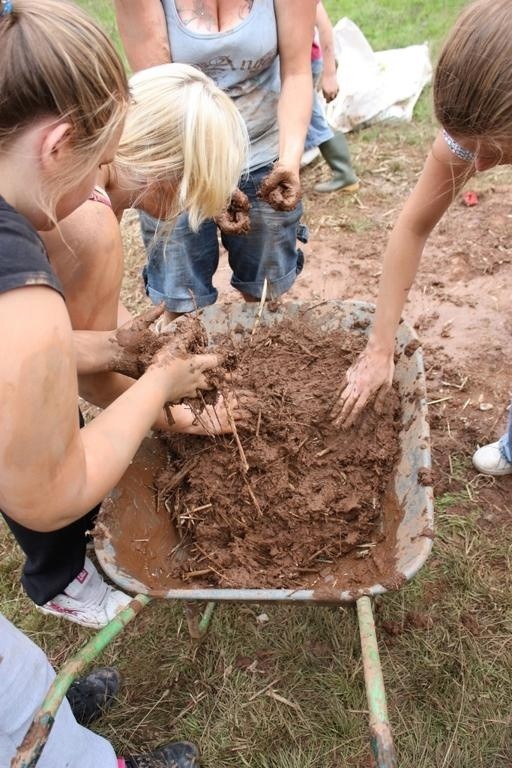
[304,2,360,194]
[331,1,511,475]
[112,1,317,327]
[1,615,200,768]
[2,0,225,631]
[32,62,260,435]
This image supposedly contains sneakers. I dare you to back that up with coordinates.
[36,556,133,629]
[66,668,119,724]
[124,743,199,768]
[473,440,512,475]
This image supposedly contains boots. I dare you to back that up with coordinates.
[313,131,358,195]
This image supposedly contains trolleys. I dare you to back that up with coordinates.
[10,302,436,768]
